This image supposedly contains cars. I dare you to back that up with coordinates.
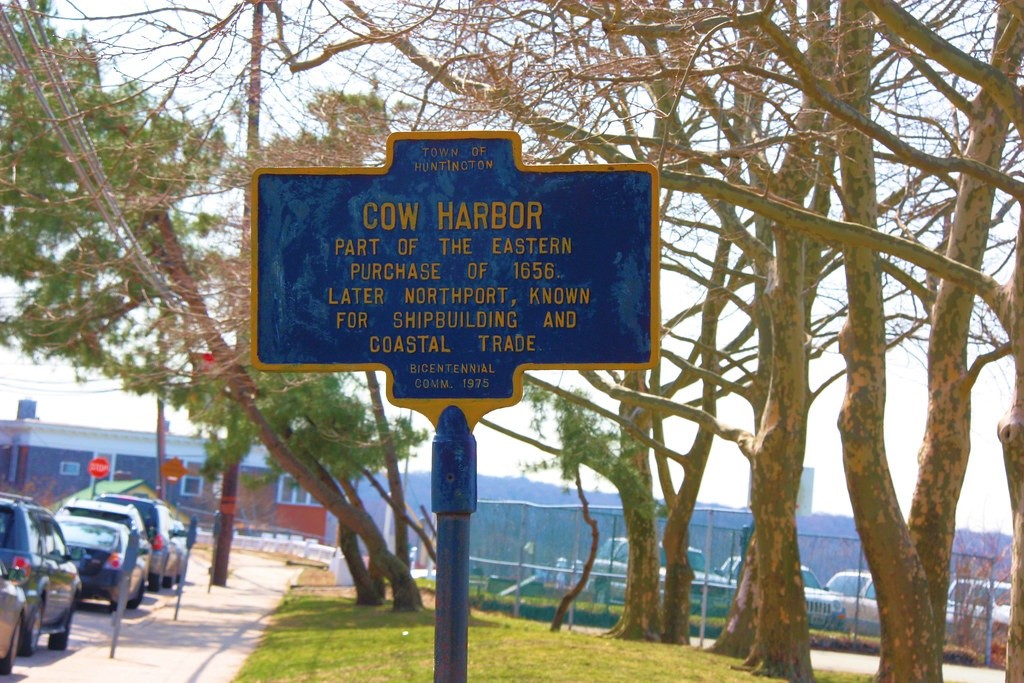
[714,555,845,631]
[0,491,85,657]
[0,560,28,677]
[587,537,737,617]
[947,579,1011,633]
[173,520,189,583]
[826,572,881,634]
[54,515,149,612]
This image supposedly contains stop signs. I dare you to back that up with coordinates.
[89,458,110,478]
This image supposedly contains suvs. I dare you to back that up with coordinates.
[56,496,153,566]
[94,493,182,591]
